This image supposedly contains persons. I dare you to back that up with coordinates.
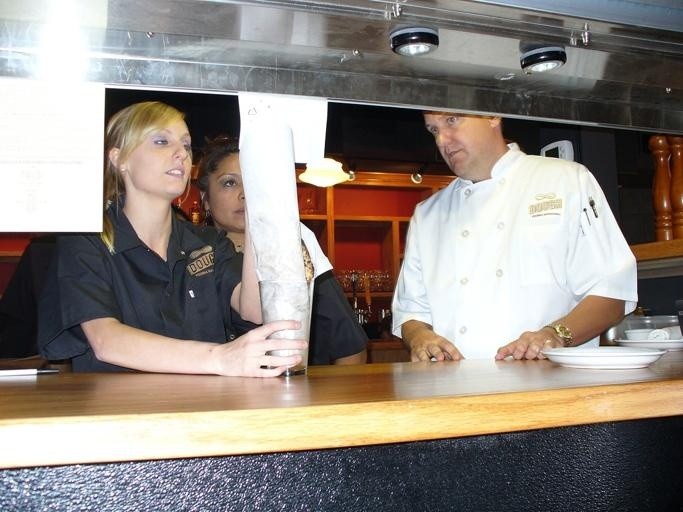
[390,109,638,361]
[1,238,56,356]
[197,136,371,366]
[37,101,309,378]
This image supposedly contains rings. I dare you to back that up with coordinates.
[544,338,555,346]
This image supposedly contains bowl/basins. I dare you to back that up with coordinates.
[624,328,653,339]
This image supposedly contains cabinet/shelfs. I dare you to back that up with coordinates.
[297,184,457,299]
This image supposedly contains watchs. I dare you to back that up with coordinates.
[547,321,574,348]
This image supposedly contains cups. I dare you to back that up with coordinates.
[255,278,311,377]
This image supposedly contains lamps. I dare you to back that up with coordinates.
[390,27,439,57]
[520,47,566,73]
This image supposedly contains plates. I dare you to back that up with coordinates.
[539,345,667,369]
[612,339,683,352]
